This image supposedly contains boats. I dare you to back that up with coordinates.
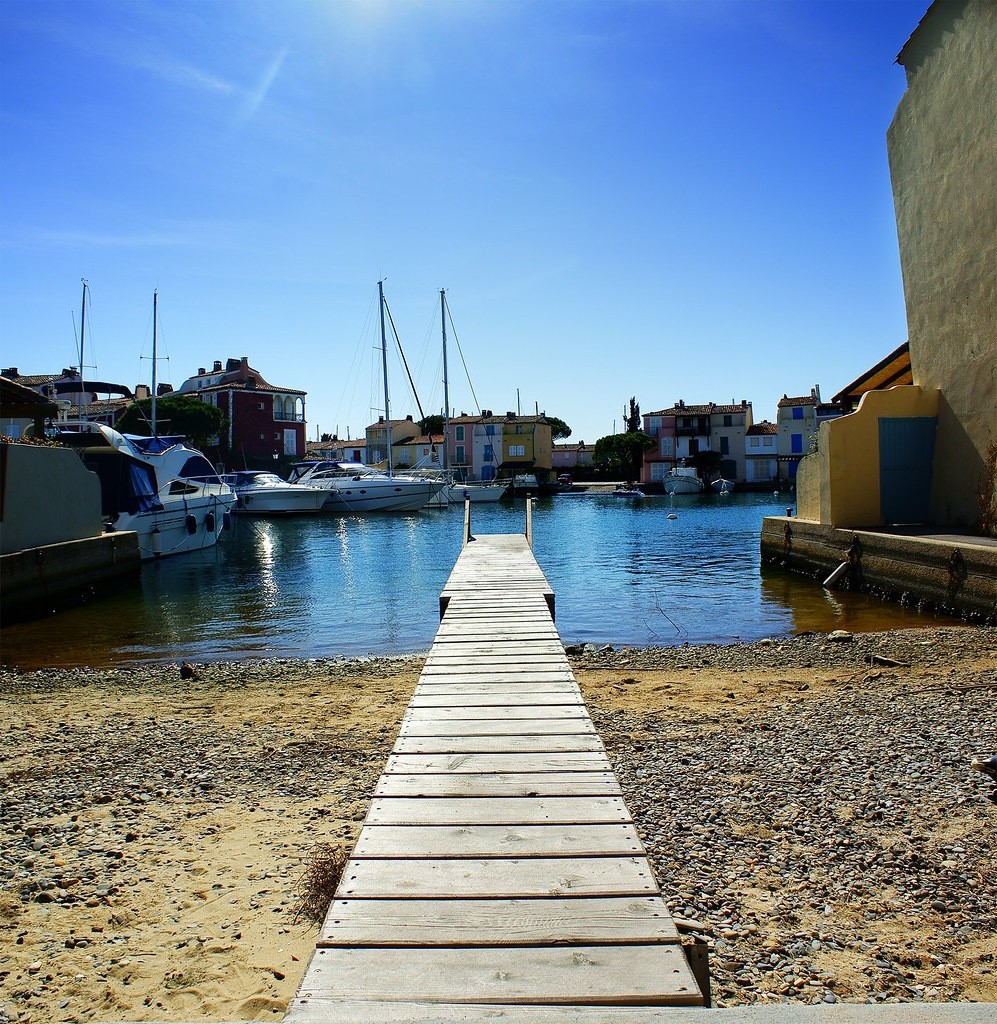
[711,474,736,495]
[661,458,705,495]
[43,279,238,560]
[513,473,541,500]
[216,444,337,514]
[610,484,646,499]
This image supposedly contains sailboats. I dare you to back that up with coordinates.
[285,278,456,513]
[428,287,511,504]
